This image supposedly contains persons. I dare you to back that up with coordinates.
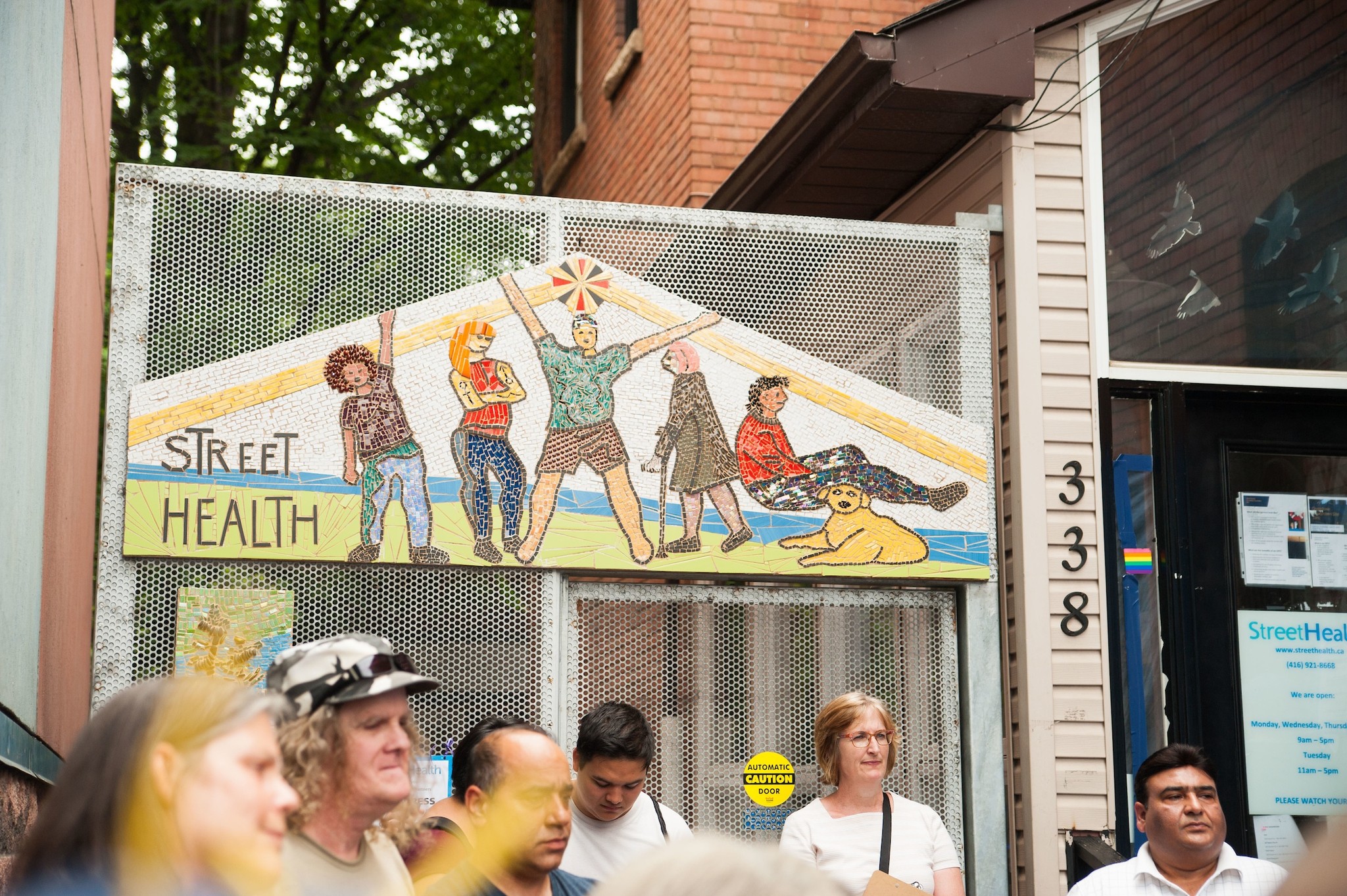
[2,632,1347,896]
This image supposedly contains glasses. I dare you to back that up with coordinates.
[308,653,417,717]
[837,728,895,748]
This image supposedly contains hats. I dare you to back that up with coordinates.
[266,635,441,717]
